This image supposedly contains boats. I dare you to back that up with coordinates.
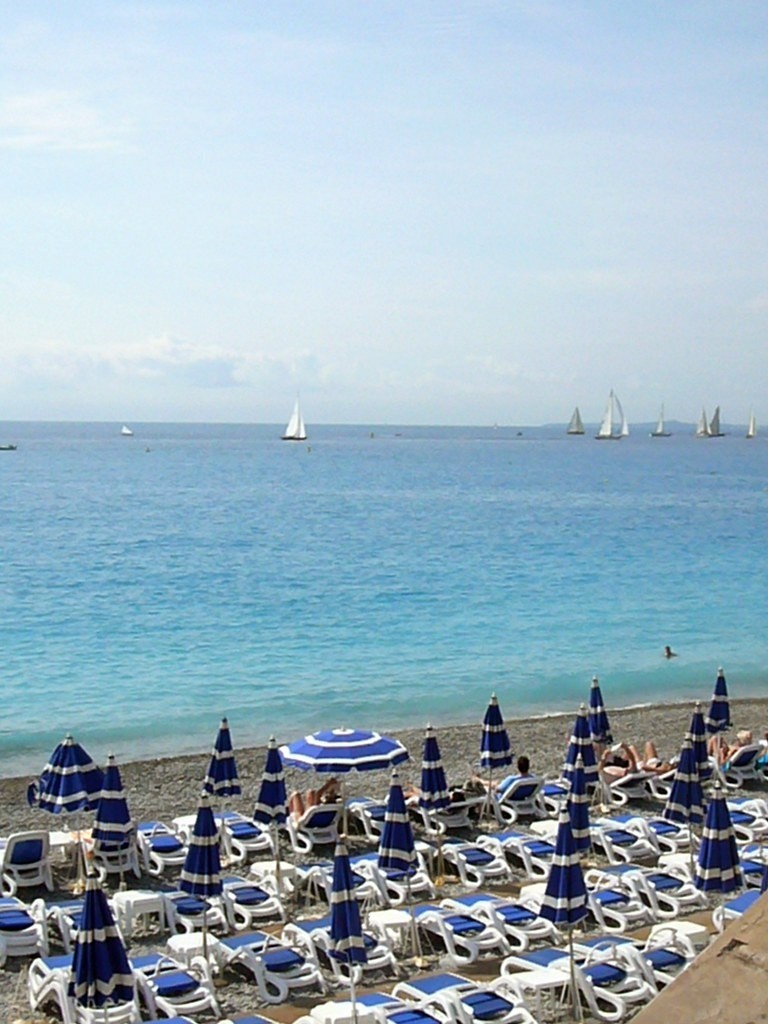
[122,425,134,435]
[0,444,17,450]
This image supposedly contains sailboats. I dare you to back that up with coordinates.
[593,387,630,440]
[566,407,585,434]
[709,405,725,437]
[279,391,308,441]
[745,403,757,439]
[696,409,712,437]
[649,403,672,437]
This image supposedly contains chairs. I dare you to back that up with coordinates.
[0,742,768,1024]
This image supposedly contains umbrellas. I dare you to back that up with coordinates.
[418,722,454,838]
[67,860,135,1022]
[27,734,106,816]
[176,789,224,959]
[693,780,743,934]
[538,753,595,1020]
[90,754,135,881]
[201,717,242,827]
[378,767,421,958]
[326,841,368,1023]
[704,667,733,753]
[561,702,599,789]
[588,676,613,744]
[479,692,513,823]
[661,732,704,865]
[253,734,289,857]
[685,699,707,769]
[278,726,415,810]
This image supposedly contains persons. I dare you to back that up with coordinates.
[597,741,680,785]
[288,777,340,821]
[494,755,535,800]
[403,781,466,813]
[706,730,768,765]
[664,645,679,660]
